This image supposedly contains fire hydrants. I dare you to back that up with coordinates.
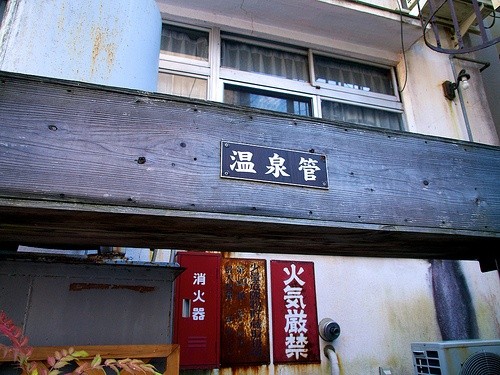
[171,250,222,370]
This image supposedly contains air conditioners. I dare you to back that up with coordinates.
[410,339,500,375]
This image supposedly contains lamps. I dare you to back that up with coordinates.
[442,68,471,100]
